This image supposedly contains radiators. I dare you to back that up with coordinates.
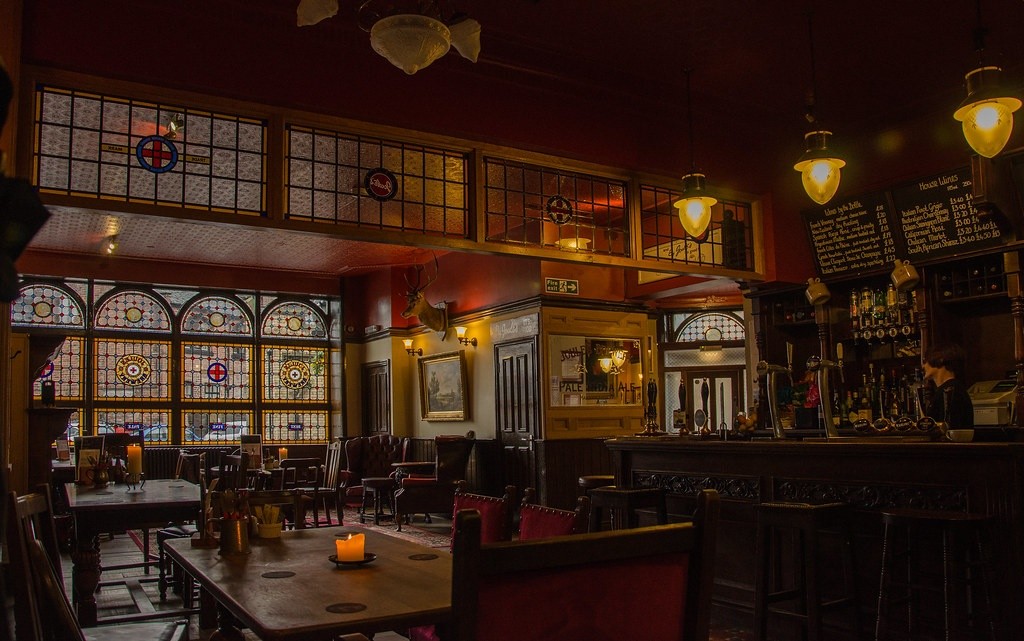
[145,446,232,480]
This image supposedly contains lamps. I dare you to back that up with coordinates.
[673,67,718,238]
[952,1,1022,158]
[402,339,423,356]
[610,344,629,373]
[556,237,593,250]
[107,234,119,253]
[793,2,846,204]
[597,346,613,374]
[164,114,184,139]
[454,326,478,347]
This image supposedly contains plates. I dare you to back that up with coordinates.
[328,553,378,566]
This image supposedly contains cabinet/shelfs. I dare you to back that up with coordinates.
[770,254,1012,421]
[26,405,77,495]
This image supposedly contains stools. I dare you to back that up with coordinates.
[752,499,857,640]
[587,485,666,534]
[873,511,995,641]
[579,475,615,532]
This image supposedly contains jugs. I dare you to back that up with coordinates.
[891,259,920,290]
[805,277,830,305]
[206,517,253,555]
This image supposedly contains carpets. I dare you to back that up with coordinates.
[127,509,452,563]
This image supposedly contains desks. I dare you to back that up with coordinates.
[64,479,218,629]
[211,462,325,529]
[361,478,397,525]
[390,462,435,522]
[50,454,123,474]
[162,525,453,641]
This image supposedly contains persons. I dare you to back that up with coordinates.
[921,343,975,441]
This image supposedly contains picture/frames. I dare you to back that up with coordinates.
[419,349,469,424]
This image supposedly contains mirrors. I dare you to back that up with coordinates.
[546,332,644,407]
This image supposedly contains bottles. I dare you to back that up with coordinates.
[849,284,917,330]
[832,363,925,429]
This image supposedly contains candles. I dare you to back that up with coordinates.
[127,446,142,475]
[335,533,365,562]
[278,447,287,462]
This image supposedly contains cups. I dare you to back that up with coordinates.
[56,440,69,460]
[945,429,974,442]
[258,523,282,538]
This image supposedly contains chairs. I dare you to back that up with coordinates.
[6,431,723,641]
